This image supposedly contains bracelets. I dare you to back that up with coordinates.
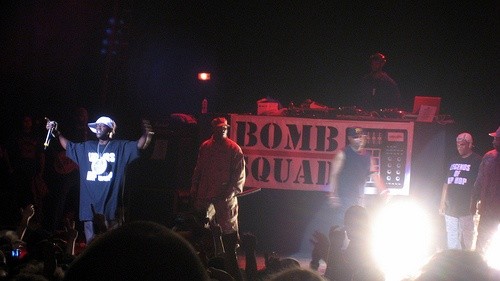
[330,197,339,200]
[39,169,44,172]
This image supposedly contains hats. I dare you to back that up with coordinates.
[368,53,387,63]
[489,127,500,137]
[212,118,231,127]
[456,133,473,142]
[348,128,368,136]
[88,116,116,134]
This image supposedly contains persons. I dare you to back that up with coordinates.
[329,128,387,225]
[440,133,483,253]
[0,206,500,281]
[352,52,400,113]
[62,107,89,142]
[6,112,46,205]
[46,116,154,243]
[190,117,246,260]
[469,128,500,251]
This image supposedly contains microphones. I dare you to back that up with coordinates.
[43,121,56,149]
